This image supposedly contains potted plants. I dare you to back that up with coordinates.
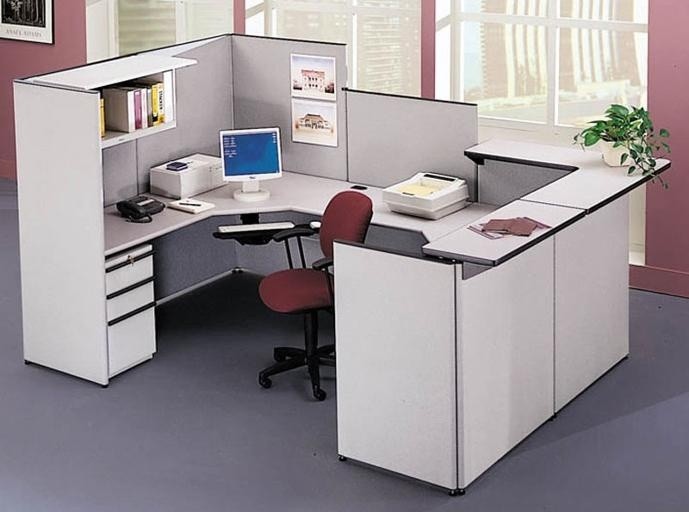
[573,103,671,190]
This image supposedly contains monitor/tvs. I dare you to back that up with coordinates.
[220,127,282,202]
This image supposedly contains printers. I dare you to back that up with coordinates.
[382,172,469,220]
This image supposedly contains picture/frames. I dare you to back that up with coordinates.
[1,0,56,45]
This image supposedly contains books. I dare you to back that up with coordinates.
[168,197,214,216]
[100,78,165,138]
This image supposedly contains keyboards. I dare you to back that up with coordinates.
[218,221,295,234]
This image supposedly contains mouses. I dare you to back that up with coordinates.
[310,221,321,229]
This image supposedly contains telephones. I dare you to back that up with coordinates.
[116,195,166,220]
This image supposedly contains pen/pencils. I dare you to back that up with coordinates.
[180,203,202,206]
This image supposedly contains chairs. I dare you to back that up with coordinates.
[259,190,375,400]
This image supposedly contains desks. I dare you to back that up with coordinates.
[105,170,521,495]
